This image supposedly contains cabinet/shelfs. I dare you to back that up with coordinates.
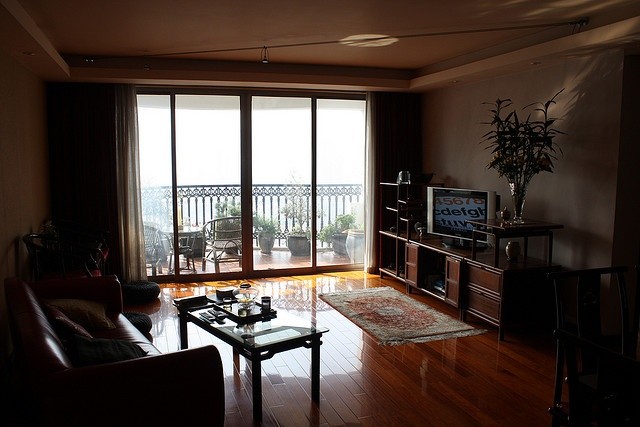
[405,241,464,320]
[463,252,561,341]
[380,181,444,292]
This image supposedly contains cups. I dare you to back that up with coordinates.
[237,303,247,317]
[223,297,231,308]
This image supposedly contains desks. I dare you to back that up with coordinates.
[465,218,563,268]
[161,225,202,275]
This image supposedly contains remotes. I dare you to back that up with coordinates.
[208,309,226,320]
[200,311,216,321]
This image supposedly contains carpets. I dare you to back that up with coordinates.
[317,285,488,346]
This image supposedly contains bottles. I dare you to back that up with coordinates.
[261,296,271,312]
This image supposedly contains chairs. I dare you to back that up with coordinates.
[203,216,241,274]
[23,233,106,281]
[143,226,164,276]
[546,265,640,426]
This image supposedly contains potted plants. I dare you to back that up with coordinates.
[254,214,280,254]
[214,201,241,255]
[320,214,360,254]
[281,173,321,255]
[346,204,365,262]
[483,88,567,225]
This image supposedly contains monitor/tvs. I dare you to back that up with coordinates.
[427,186,501,252]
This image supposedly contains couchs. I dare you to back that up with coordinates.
[4,274,225,426]
